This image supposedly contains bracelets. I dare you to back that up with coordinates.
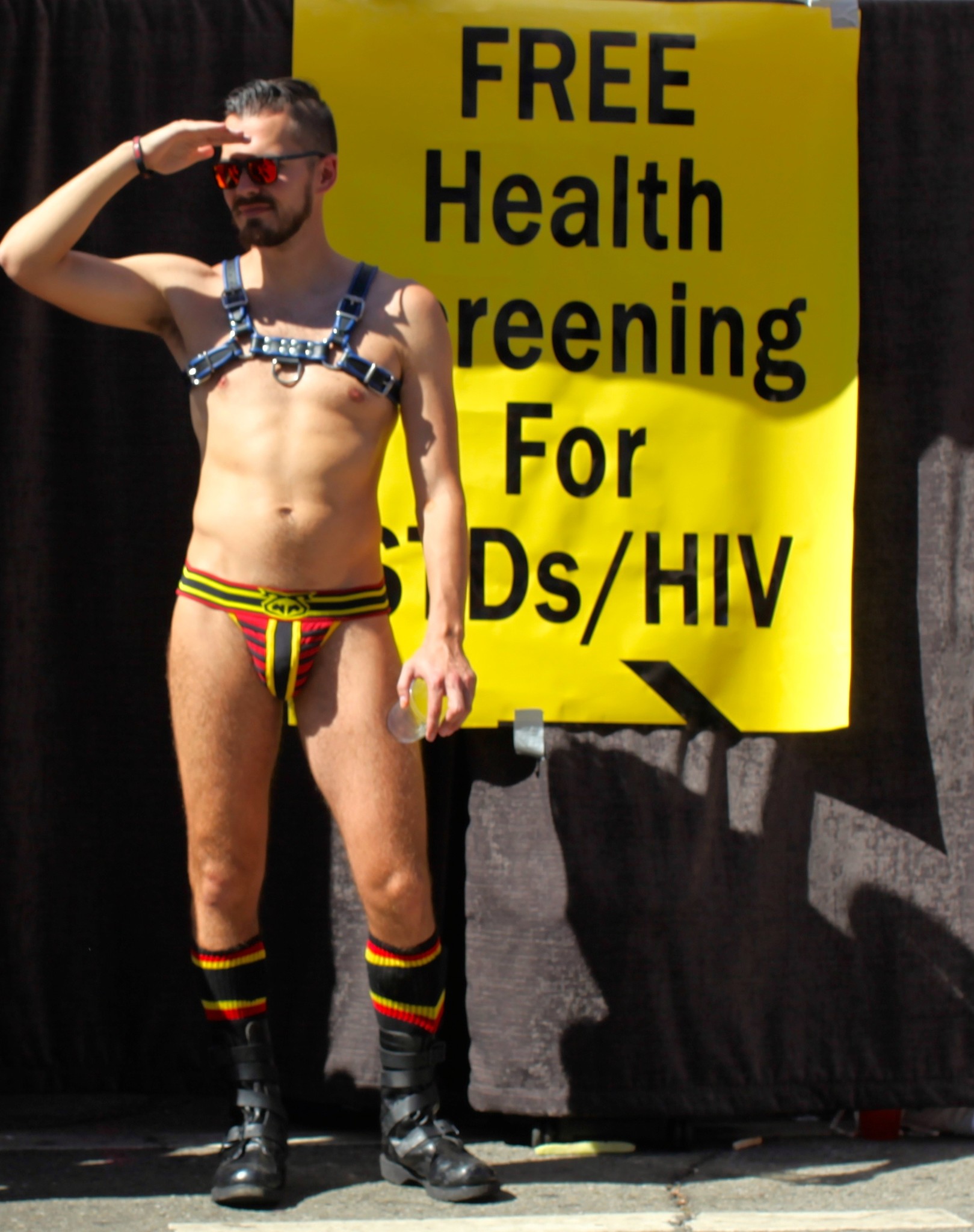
[133,135,151,180]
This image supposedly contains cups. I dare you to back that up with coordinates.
[387,680,449,743]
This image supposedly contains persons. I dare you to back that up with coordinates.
[0,78,499,1208]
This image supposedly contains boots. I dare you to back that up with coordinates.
[211,1017,287,1204]
[378,1022,500,1202]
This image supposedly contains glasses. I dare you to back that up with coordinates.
[212,151,326,190]
